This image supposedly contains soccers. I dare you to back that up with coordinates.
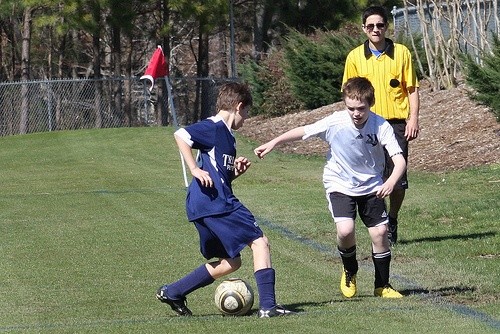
[214,277,254,317]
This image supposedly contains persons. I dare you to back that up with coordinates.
[254,77,407,298]
[341,7,420,249]
[155,83,304,319]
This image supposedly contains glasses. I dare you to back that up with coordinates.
[362,23,386,29]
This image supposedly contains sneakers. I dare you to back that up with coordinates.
[340,265,358,297]
[386,213,398,246]
[374,284,403,298]
[156,284,192,316]
[257,305,300,319]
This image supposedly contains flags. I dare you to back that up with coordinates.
[140,45,168,92]
